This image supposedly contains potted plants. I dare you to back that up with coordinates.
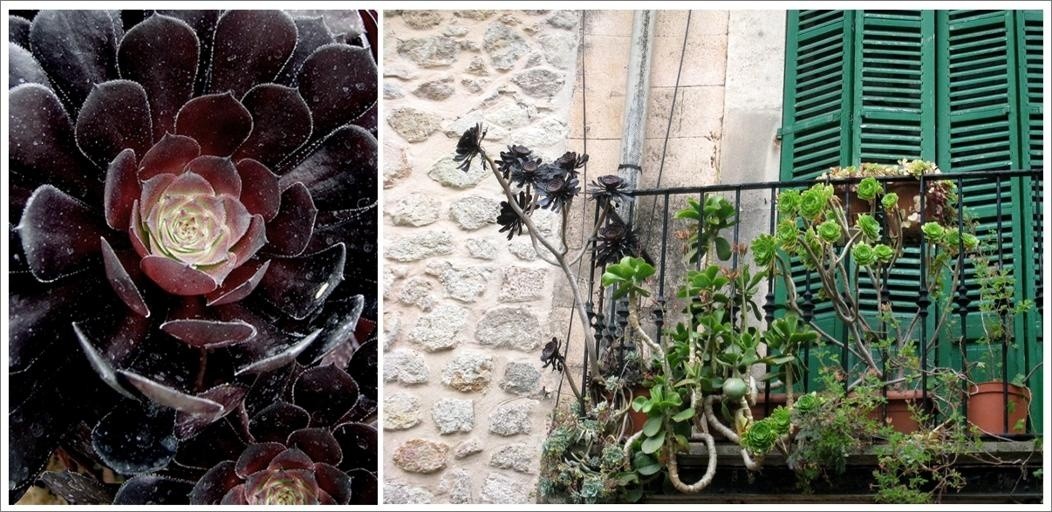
[453,126,1035,504]
